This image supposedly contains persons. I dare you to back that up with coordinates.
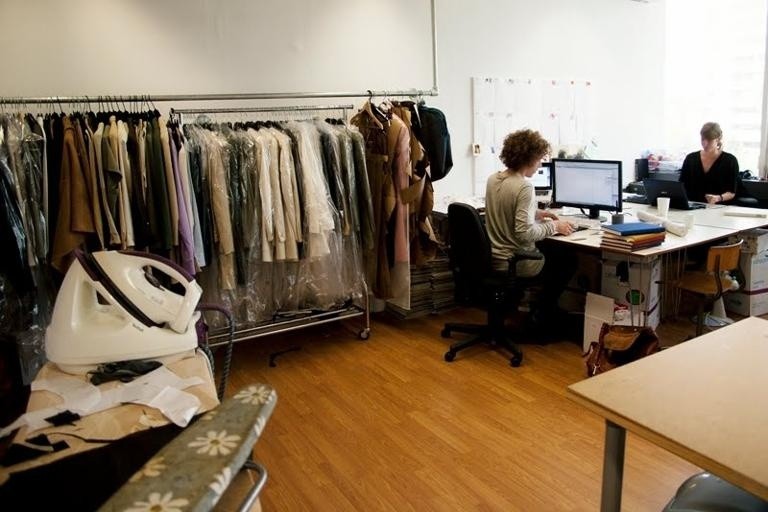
[484,129,574,317]
[678,122,743,205]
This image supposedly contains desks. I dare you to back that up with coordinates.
[429,187,768,266]
[564,313,768,511]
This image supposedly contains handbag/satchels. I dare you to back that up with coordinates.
[582,322,662,376]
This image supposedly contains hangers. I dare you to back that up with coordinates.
[168,102,361,165]
[0,88,164,165]
[350,88,445,165]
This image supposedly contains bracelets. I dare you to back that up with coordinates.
[720,193,724,201]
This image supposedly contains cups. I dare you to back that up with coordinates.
[611,214,624,225]
[657,198,670,220]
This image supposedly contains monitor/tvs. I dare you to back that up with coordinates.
[523,162,553,190]
[552,159,623,222]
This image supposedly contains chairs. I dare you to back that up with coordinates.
[439,201,549,368]
[669,241,747,327]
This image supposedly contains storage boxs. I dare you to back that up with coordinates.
[724,229,768,316]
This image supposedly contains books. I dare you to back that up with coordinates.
[600,223,666,254]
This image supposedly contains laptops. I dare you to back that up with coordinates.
[643,178,707,210]
